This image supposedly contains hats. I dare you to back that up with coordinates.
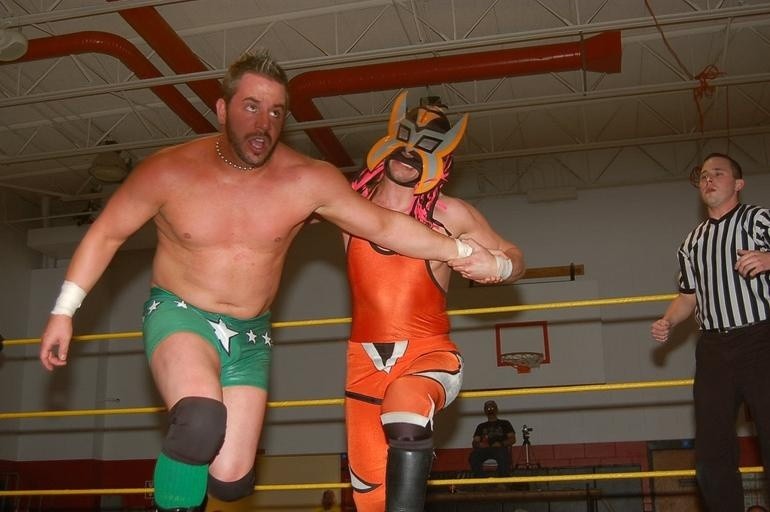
[485,402,497,408]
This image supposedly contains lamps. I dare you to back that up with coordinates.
[88,138,130,185]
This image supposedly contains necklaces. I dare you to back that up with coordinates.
[212,134,256,174]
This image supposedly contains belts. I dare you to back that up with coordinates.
[703,325,747,337]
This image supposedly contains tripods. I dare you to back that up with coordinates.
[516,437,541,469]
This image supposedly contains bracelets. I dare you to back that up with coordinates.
[49,280,87,318]
[453,237,473,259]
[493,253,513,281]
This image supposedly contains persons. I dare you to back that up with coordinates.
[32,50,512,512]
[646,151,770,511]
[468,399,517,477]
[309,488,341,512]
[300,94,530,511]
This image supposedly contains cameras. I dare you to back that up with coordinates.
[523,426,532,435]
